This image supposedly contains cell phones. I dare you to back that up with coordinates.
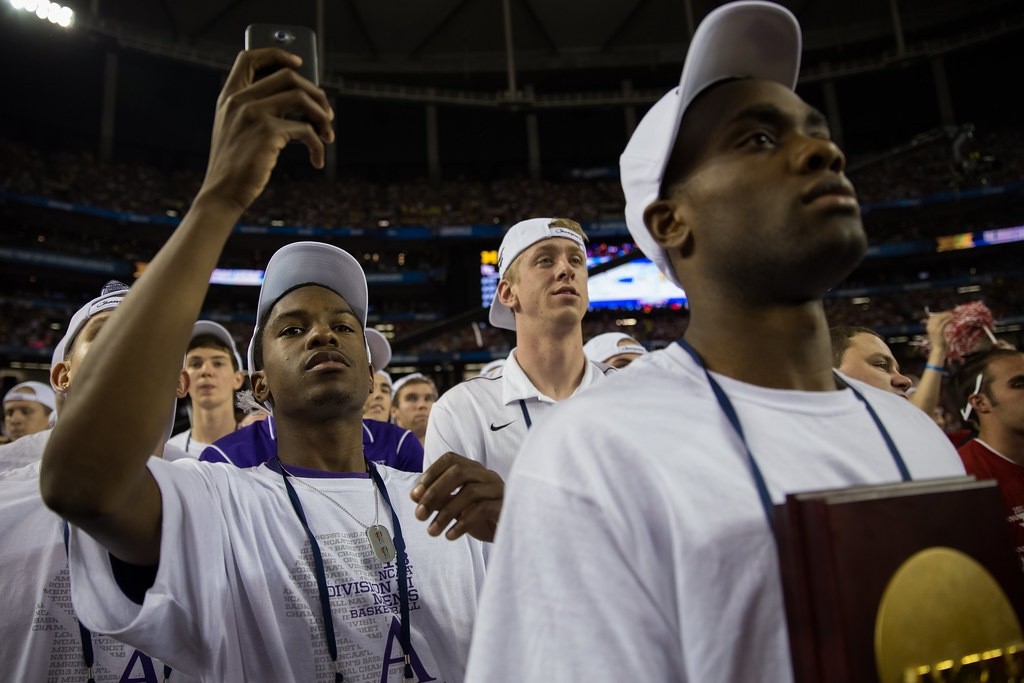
[250,27,320,136]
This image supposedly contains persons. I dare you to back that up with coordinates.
[423,216,629,480]
[0,0,1024,683]
[460,1,969,683]
[40,46,504,683]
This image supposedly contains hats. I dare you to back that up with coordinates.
[389,373,438,424]
[365,328,392,373]
[188,318,242,373]
[489,218,588,331]
[50,290,185,444]
[248,241,372,415]
[620,1,802,288]
[3,381,58,430]
[582,332,647,361]
